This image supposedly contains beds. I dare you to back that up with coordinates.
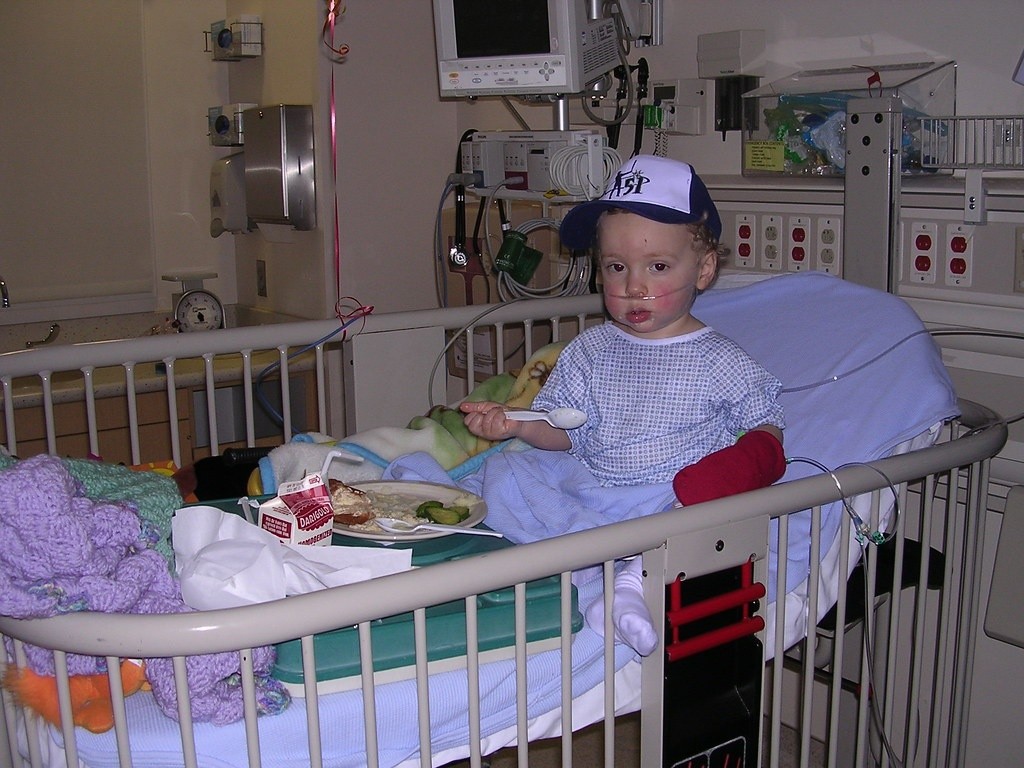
[14,250,949,768]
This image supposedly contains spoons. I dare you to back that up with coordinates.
[374,517,504,539]
[503,408,589,429]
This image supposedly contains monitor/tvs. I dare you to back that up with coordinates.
[431,0,621,97]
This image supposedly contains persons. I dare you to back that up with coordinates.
[460,154,785,657]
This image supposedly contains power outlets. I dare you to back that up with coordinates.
[788,216,811,272]
[734,213,756,267]
[908,221,939,285]
[758,215,784,270]
[1014,227,1024,294]
[944,223,976,287]
[255,258,267,298]
[815,216,842,274]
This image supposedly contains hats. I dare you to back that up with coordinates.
[560,154,722,255]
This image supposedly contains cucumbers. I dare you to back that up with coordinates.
[417,501,470,525]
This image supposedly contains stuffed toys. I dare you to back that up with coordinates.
[2,657,153,734]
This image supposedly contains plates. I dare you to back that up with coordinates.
[333,480,488,543]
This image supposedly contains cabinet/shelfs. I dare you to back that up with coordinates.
[0,370,318,473]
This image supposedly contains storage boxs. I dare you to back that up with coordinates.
[741,56,959,181]
